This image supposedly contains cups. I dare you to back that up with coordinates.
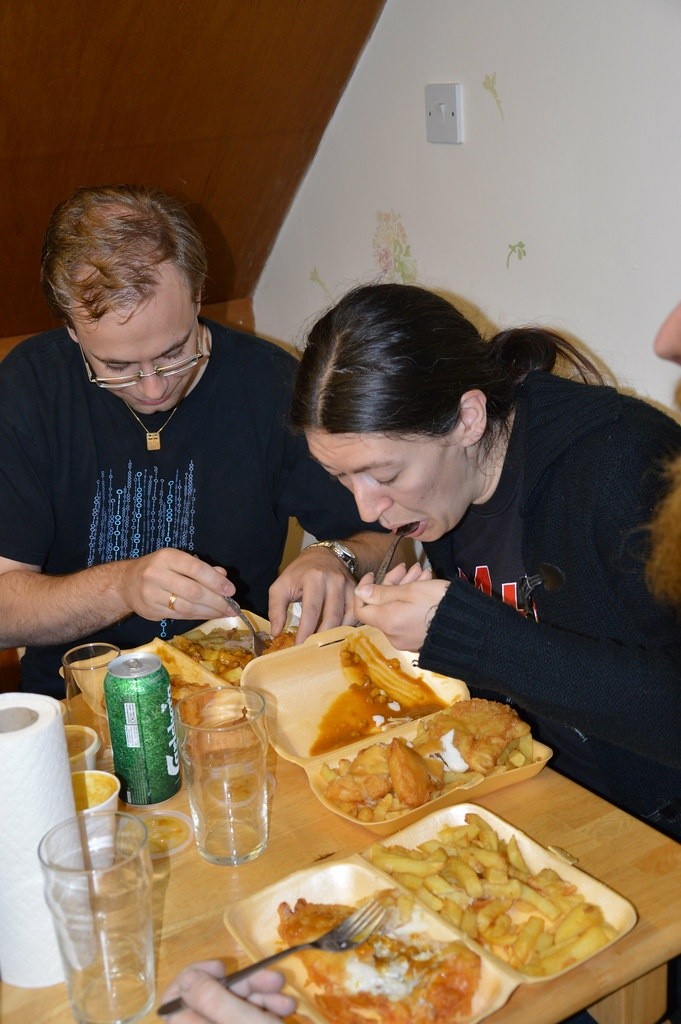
[38,810,157,1024]
[61,642,123,773]
[172,685,269,867]
[70,770,120,879]
[64,724,101,774]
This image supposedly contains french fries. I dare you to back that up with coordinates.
[370,810,620,975]
[316,711,531,823]
[184,629,244,686]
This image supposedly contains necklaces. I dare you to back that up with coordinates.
[123,398,177,452]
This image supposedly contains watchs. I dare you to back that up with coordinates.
[305,540,359,576]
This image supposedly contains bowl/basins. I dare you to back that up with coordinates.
[223,802,638,1024]
[239,624,553,838]
[58,610,297,740]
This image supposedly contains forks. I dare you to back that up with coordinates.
[155,897,387,1019]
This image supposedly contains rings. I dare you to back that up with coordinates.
[169,594,177,611]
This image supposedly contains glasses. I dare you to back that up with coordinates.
[78,297,206,391]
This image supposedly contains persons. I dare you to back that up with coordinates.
[0,184,418,702]
[288,285,681,1024]
[160,959,296,1024]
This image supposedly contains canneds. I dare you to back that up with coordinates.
[102,652,182,808]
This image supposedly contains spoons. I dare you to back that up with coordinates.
[193,554,269,658]
[372,521,420,584]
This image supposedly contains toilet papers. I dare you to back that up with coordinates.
[1,691,98,991]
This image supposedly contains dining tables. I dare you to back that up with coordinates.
[0,691,680,1023]
